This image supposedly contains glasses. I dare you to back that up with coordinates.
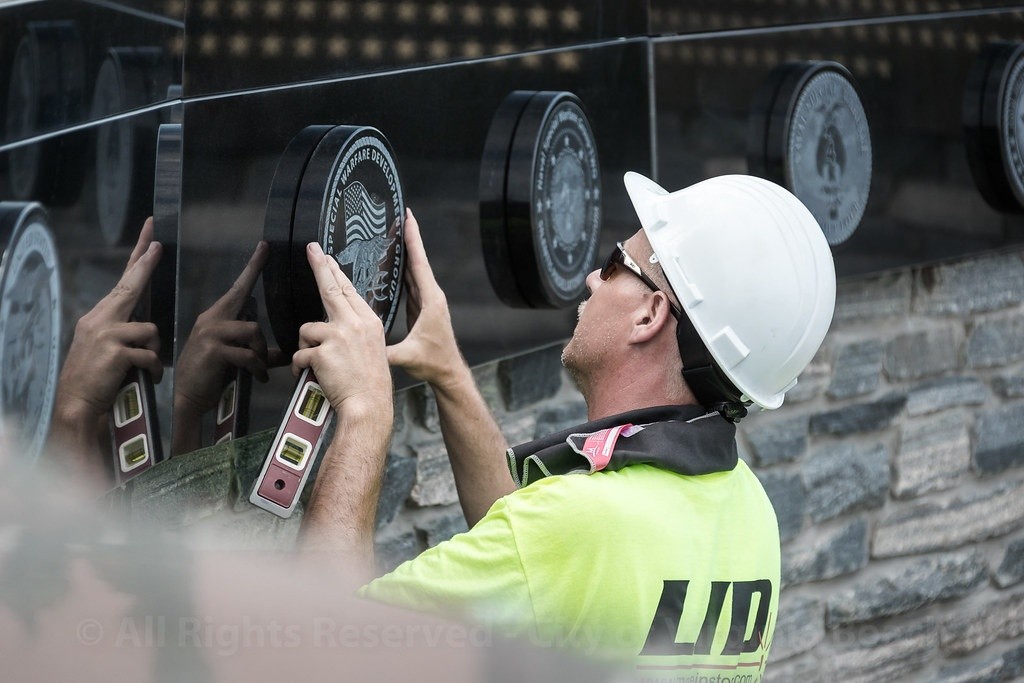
[599,241,682,324]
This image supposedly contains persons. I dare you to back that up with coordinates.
[46,215,166,503]
[294,171,836,683]
[168,237,268,465]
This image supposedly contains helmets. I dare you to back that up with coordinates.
[624,169,839,411]
